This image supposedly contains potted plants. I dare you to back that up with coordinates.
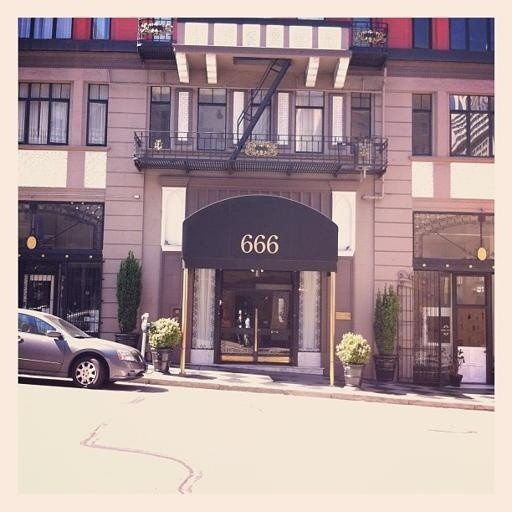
[335,331,371,387]
[372,283,398,381]
[414,343,464,387]
[148,317,182,372]
[114,250,143,349]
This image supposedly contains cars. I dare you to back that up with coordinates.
[66,312,97,332]
[18,308,148,389]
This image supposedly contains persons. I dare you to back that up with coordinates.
[242,314,252,347]
[236,315,245,347]
